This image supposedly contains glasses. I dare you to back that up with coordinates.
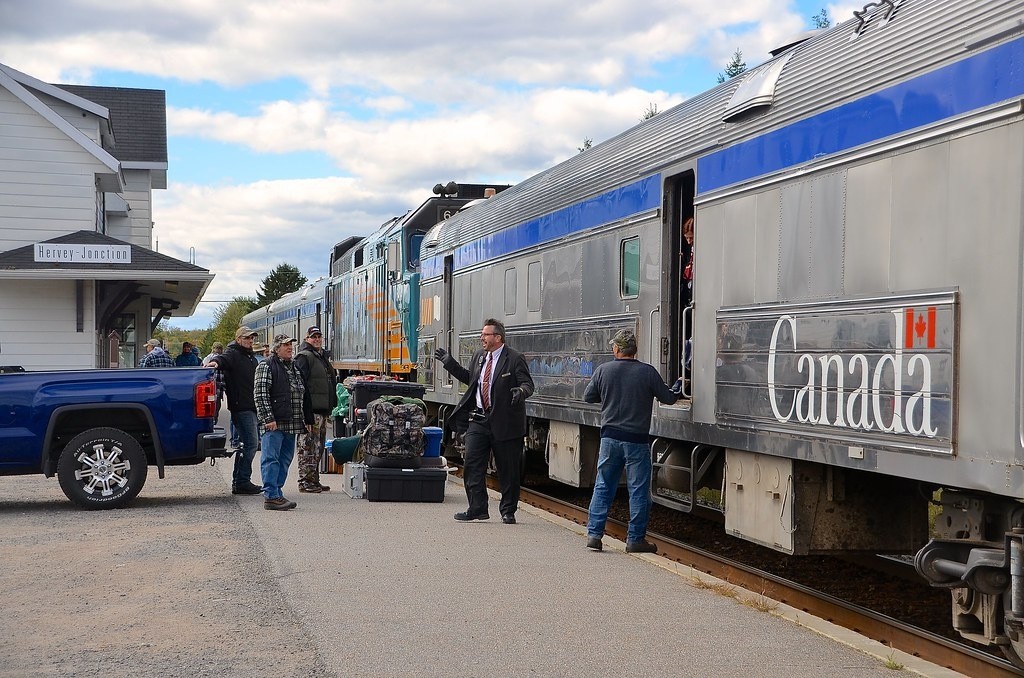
[611,343,614,349]
[480,333,500,337]
[312,336,321,339]
[245,337,254,340]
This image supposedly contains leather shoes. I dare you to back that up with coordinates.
[454,510,489,520]
[502,513,516,523]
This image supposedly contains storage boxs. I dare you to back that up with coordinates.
[421,426,444,456]
[342,462,364,499]
[351,381,425,422]
[330,416,344,437]
[364,469,447,503]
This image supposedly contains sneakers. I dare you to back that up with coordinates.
[264,497,296,509]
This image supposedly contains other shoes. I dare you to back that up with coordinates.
[232,485,262,494]
[300,483,330,493]
[626,541,658,552]
[587,537,602,550]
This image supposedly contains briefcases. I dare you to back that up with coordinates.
[342,462,363,499]
[320,447,343,473]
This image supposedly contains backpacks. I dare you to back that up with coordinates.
[351,394,428,468]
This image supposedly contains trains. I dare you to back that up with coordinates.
[239,181,514,383]
[417,0,1024,671]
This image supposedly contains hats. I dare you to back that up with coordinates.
[183,342,192,350]
[608,330,635,344]
[192,348,200,353]
[235,326,258,338]
[274,334,297,344]
[683,218,694,237]
[252,342,266,351]
[143,339,161,347]
[308,326,323,337]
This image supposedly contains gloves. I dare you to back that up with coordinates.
[435,346,453,364]
[510,387,528,408]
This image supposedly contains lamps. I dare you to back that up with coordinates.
[164,281,178,289]
[162,298,173,308]
[163,312,171,319]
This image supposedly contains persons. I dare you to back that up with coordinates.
[203,326,262,495]
[433,319,534,524]
[684,218,695,283]
[293,326,336,493]
[583,330,690,554]
[254,333,314,511]
[138,338,270,449]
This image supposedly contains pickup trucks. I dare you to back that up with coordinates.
[0,367,244,511]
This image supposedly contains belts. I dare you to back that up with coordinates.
[474,406,490,414]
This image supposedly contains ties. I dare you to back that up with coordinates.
[483,352,493,409]
